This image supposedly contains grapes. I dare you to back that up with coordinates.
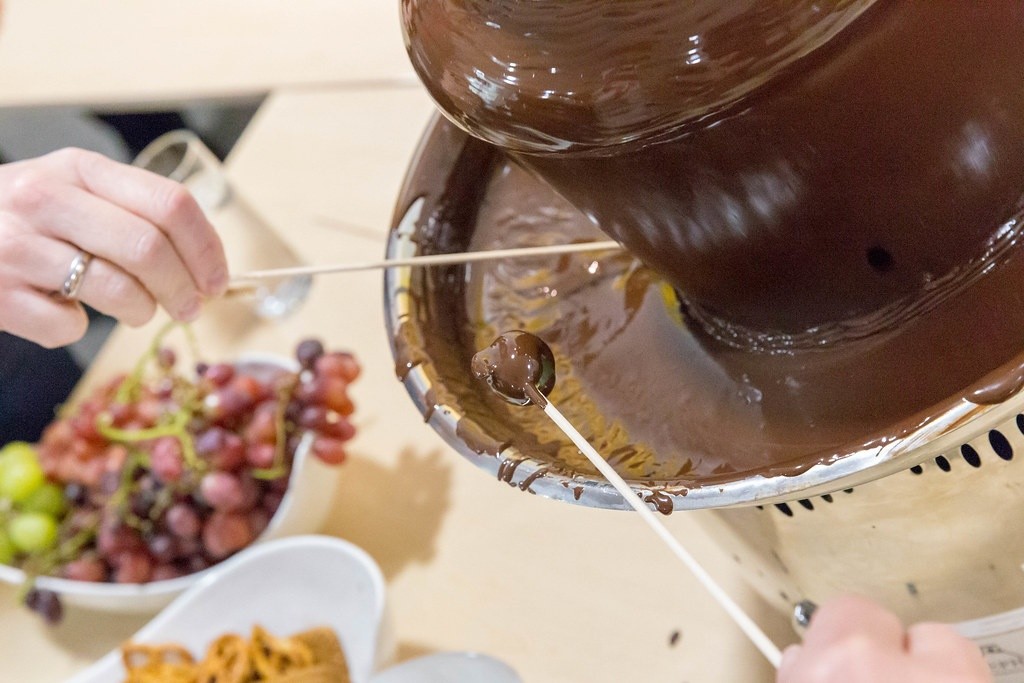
[0,339,360,585]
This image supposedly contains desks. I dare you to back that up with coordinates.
[0,0,438,163]
[1,86,1024,683]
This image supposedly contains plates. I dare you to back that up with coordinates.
[78,536,387,683]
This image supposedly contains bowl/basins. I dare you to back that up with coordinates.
[0,355,312,611]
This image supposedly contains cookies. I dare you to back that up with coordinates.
[122,622,356,683]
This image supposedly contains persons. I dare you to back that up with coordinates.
[0,143,228,447]
[776,605,995,683]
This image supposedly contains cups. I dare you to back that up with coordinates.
[128,130,312,318]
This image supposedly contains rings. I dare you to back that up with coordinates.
[60,254,92,301]
